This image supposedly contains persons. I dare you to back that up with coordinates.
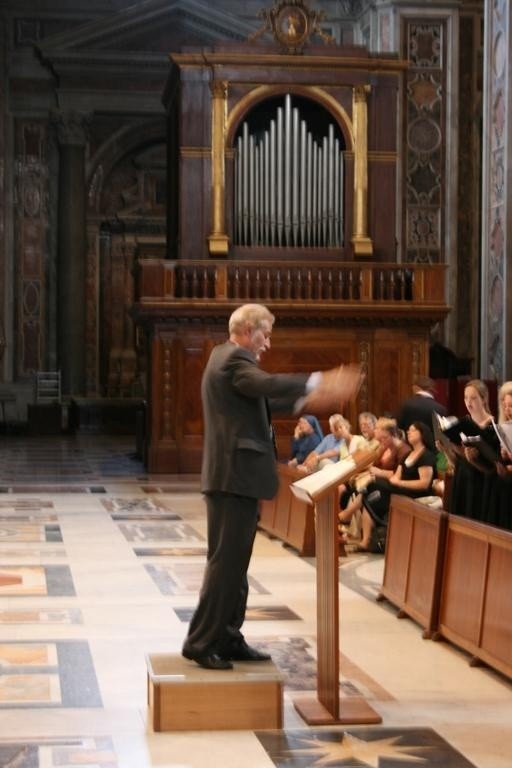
[182,303,364,669]
[289,374,511,551]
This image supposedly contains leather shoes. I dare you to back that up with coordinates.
[226,637,270,661]
[182,648,233,670]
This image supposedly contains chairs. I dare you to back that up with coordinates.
[356,427,461,552]
